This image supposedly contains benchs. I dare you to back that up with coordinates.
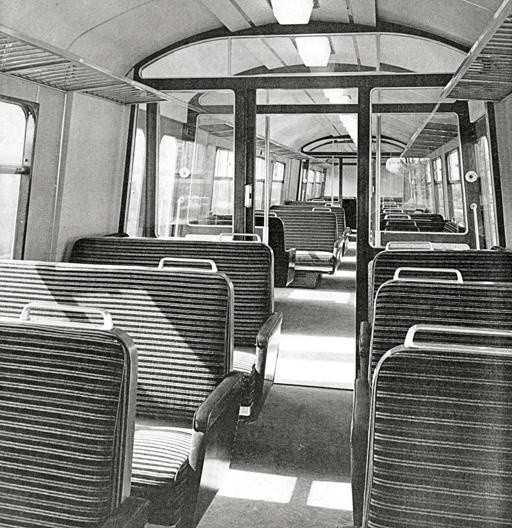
[0,234,280,527]
[349,240,512,528]
[254,196,459,288]
